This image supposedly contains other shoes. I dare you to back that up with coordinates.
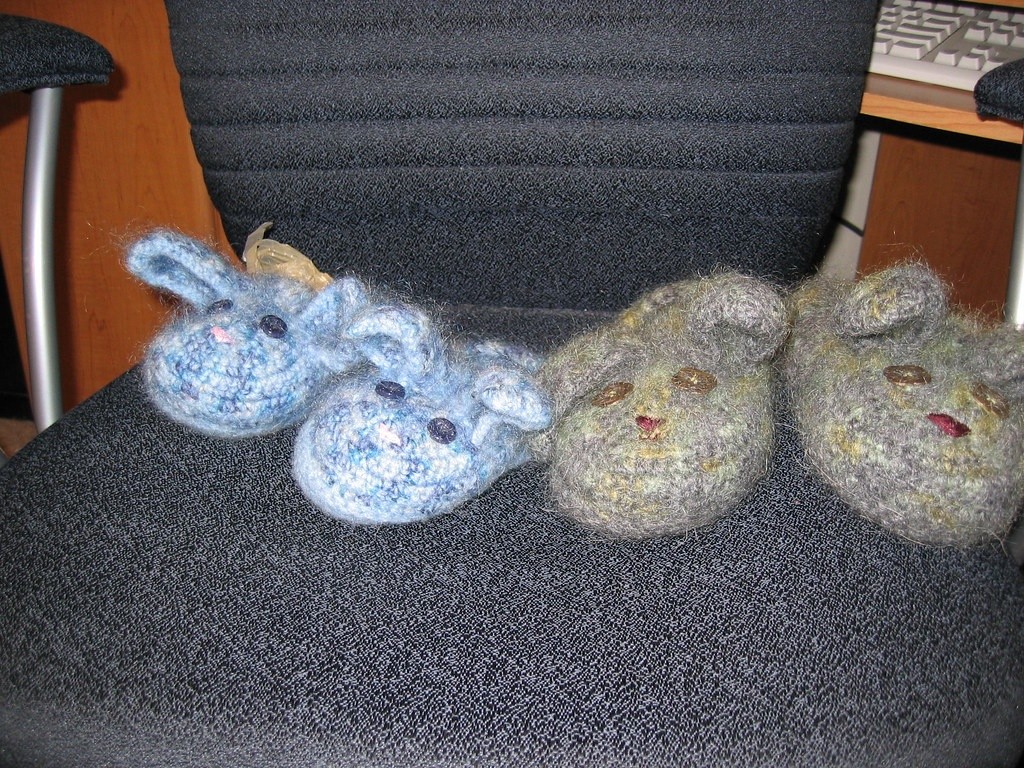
[294,301,549,526]
[128,229,365,442]
[540,273,790,534]
[797,267,1022,546]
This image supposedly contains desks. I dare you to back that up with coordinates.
[860,1,1024,322]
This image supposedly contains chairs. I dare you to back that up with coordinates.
[0,0,1024,768]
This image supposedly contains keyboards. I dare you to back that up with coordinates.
[867,0,1024,91]
